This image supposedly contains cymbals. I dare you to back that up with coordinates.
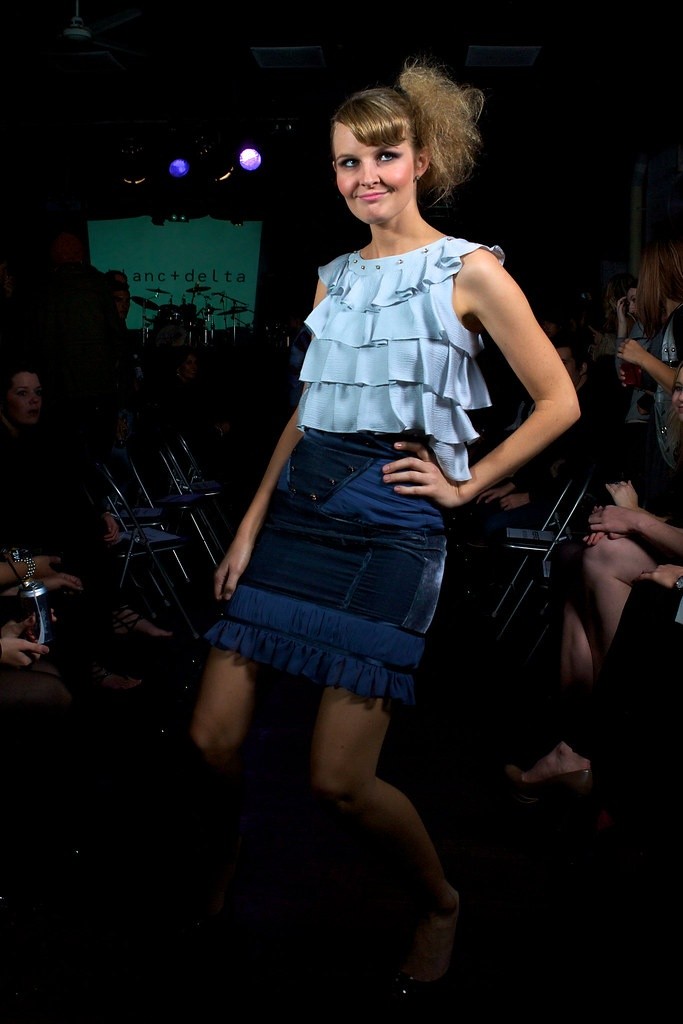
[217,307,248,316]
[146,288,171,295]
[130,295,159,311]
[186,286,212,293]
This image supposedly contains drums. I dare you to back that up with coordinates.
[153,303,206,346]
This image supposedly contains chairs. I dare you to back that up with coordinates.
[489,454,604,658]
[93,428,236,641]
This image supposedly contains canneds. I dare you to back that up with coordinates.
[17,582,52,645]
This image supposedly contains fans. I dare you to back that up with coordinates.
[16,0,145,67]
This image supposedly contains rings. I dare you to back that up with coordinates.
[614,482,620,485]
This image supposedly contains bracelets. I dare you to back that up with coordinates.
[12,556,36,583]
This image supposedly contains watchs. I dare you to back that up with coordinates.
[672,575,683,593]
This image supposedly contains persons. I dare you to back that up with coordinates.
[188,62,583,1008]
[1,230,232,798]
[430,194,683,820]
[283,302,313,416]
